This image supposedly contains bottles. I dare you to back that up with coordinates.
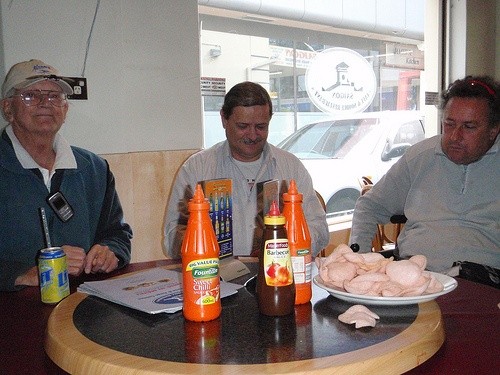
[255,198,297,318]
[181,183,222,322]
[282,180,313,307]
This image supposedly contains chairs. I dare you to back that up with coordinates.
[313,174,408,258]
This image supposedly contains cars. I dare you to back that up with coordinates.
[272,111,437,214]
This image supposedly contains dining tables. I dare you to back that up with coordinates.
[0,257,500,375]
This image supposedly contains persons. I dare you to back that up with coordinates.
[348,74,500,288]
[0,59,133,292]
[163,81,329,259]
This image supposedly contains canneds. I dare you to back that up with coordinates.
[38,246,70,303]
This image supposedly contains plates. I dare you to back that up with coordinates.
[313,271,459,306]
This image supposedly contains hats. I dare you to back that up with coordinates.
[3,59,73,98]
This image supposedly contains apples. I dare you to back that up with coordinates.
[266,261,290,282]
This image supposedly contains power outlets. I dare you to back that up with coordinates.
[67,77,87,99]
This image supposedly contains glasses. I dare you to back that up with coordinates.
[7,90,67,107]
